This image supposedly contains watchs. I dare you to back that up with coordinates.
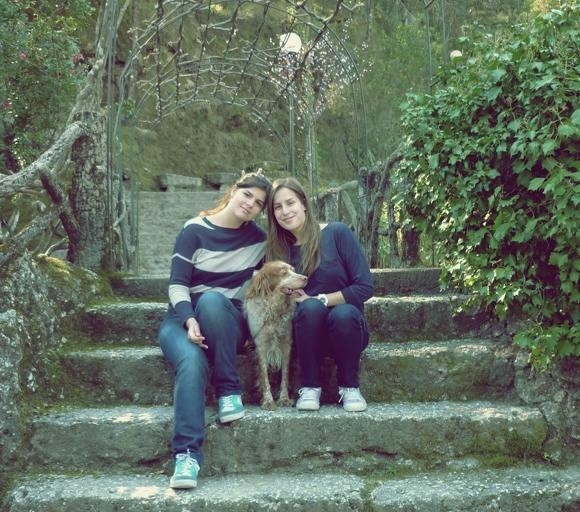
[318,293,328,307]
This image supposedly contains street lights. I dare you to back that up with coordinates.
[277,28,302,182]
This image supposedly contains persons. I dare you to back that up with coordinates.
[157,172,273,489]
[266,177,375,412]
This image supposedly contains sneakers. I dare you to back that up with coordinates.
[338,386,367,412]
[297,387,321,410]
[217,394,244,423]
[171,451,198,488]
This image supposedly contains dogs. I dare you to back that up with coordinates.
[238,260,308,411]
